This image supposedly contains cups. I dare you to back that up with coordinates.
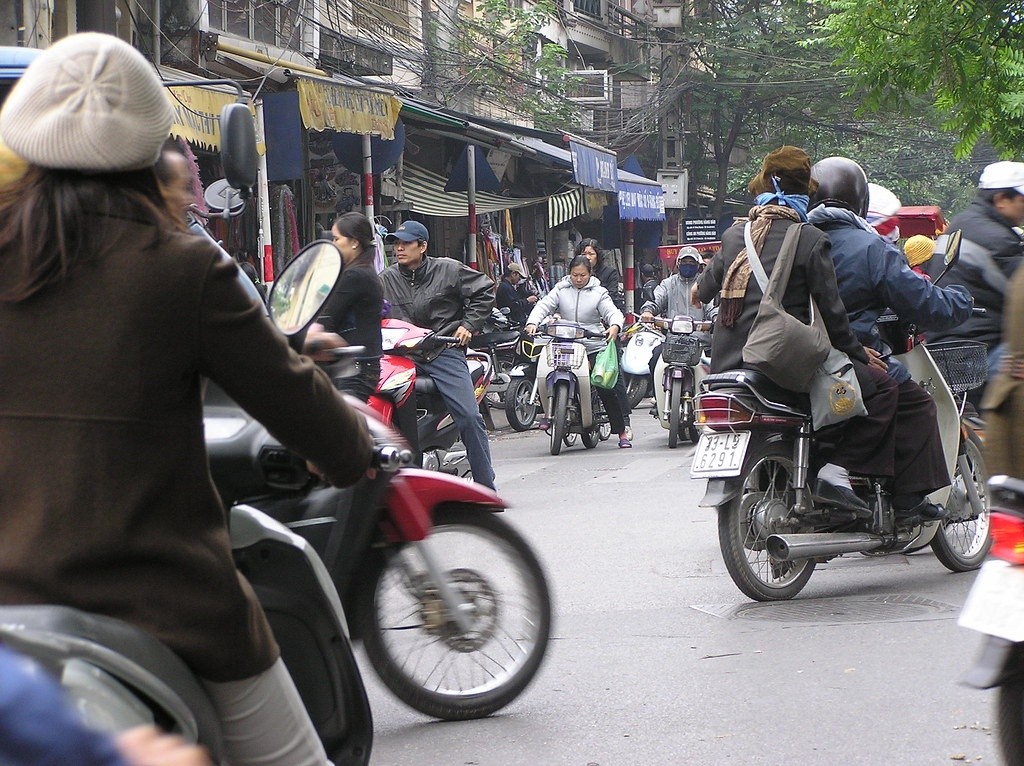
[593,366,605,382]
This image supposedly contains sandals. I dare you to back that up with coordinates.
[539,417,552,431]
[896,496,951,522]
[618,439,632,449]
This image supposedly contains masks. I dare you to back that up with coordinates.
[679,263,699,278]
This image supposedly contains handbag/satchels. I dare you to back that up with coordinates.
[742,297,831,392]
[591,336,619,389]
[809,347,869,431]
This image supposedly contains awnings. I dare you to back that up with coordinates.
[403,100,667,227]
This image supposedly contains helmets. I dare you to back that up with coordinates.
[807,156,869,219]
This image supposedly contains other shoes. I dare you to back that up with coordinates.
[649,402,658,416]
[625,426,633,441]
[814,478,873,518]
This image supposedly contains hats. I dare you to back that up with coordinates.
[676,246,707,267]
[903,235,935,267]
[384,221,429,242]
[748,146,819,198]
[508,262,528,278]
[1,31,172,171]
[641,264,654,276]
[978,161,1024,195]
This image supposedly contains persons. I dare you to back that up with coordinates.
[376,221,496,492]
[0,32,382,765]
[525,255,632,449]
[637,246,720,416]
[498,263,536,328]
[690,145,1024,518]
[574,238,634,440]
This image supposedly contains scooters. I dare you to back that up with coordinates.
[266,343,552,722]
[956,473,1024,766]
[0,237,416,766]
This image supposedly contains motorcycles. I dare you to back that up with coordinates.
[522,320,609,457]
[368,318,495,485]
[598,306,716,450]
[468,307,554,434]
[688,228,996,605]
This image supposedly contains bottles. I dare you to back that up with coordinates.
[601,366,615,385]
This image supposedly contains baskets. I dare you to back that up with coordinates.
[661,340,703,366]
[929,340,989,394]
[546,343,586,369]
